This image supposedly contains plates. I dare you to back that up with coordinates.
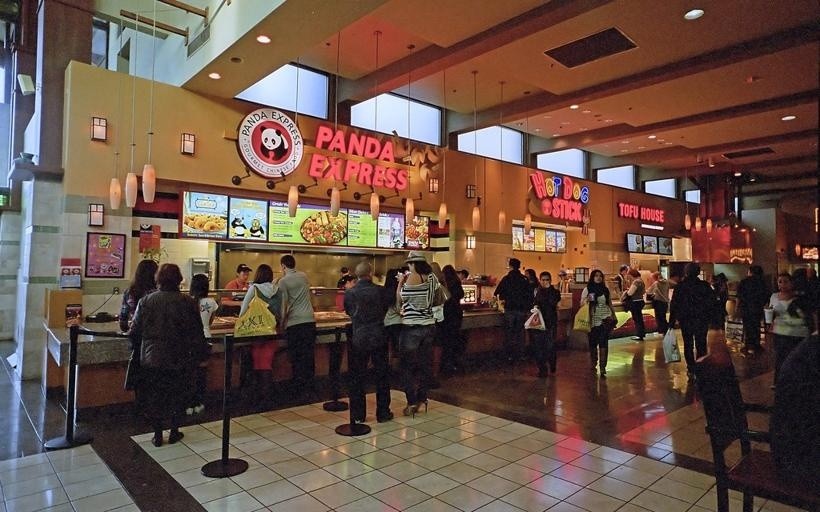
[300,211,348,243]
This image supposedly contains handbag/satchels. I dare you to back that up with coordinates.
[432,272,452,305]
[234,287,277,338]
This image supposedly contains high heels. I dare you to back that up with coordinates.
[403,405,419,419]
[416,399,428,413]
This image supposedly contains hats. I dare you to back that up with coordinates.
[457,270,468,276]
[404,252,426,264]
[237,264,252,273]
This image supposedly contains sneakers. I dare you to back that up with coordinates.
[186,407,195,415]
[194,404,205,414]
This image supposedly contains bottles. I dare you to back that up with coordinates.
[390,217,401,240]
[481,300,492,308]
[335,290,345,312]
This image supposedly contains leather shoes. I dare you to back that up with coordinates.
[169,432,184,444]
[152,437,163,447]
[377,412,394,423]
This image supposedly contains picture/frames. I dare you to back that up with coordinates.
[85,232,126,278]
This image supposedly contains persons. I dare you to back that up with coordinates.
[645,271,669,336]
[670,262,719,378]
[770,330,819,479]
[669,270,680,289]
[127,261,211,448]
[278,254,318,404]
[580,270,618,376]
[556,270,570,293]
[184,273,219,416]
[333,248,471,428]
[223,263,253,290]
[238,263,281,407]
[710,265,820,353]
[761,272,813,389]
[493,258,561,377]
[118,259,159,424]
[614,264,646,340]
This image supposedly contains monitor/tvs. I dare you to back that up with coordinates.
[460,284,477,304]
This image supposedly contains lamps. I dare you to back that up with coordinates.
[181,133,195,155]
[110,16,123,210]
[288,54,300,218]
[90,116,108,141]
[684,168,713,232]
[438,67,447,229]
[429,178,438,193]
[330,32,340,217]
[466,184,475,198]
[370,30,380,221]
[473,72,481,230]
[467,235,477,249]
[88,203,104,226]
[405,44,414,224]
[523,118,533,235]
[142,0,157,204]
[125,1,138,208]
[499,81,506,227]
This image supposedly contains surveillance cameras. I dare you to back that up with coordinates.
[17,73,35,96]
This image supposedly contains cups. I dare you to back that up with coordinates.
[588,293,596,301]
[764,309,775,324]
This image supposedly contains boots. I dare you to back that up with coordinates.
[599,348,608,374]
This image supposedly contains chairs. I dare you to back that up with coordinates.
[694,351,820,511]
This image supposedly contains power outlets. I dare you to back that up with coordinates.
[113,287,119,295]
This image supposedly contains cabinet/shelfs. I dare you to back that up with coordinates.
[41,288,83,399]
